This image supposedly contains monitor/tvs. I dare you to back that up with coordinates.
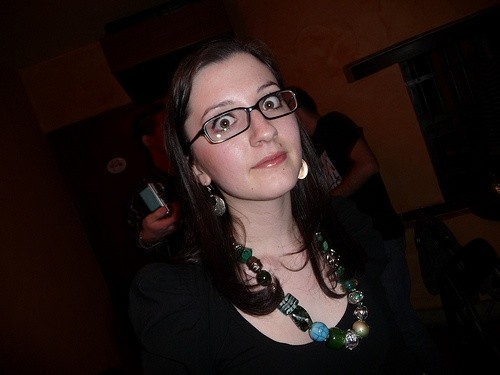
[396,6,500,204]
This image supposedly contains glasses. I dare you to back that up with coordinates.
[183,90,297,146]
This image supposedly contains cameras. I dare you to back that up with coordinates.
[139,182,173,221]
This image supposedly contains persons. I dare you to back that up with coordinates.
[283,83,437,375]
[126,95,213,282]
[142,39,393,375]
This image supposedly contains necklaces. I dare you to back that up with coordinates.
[225,231,371,351]
